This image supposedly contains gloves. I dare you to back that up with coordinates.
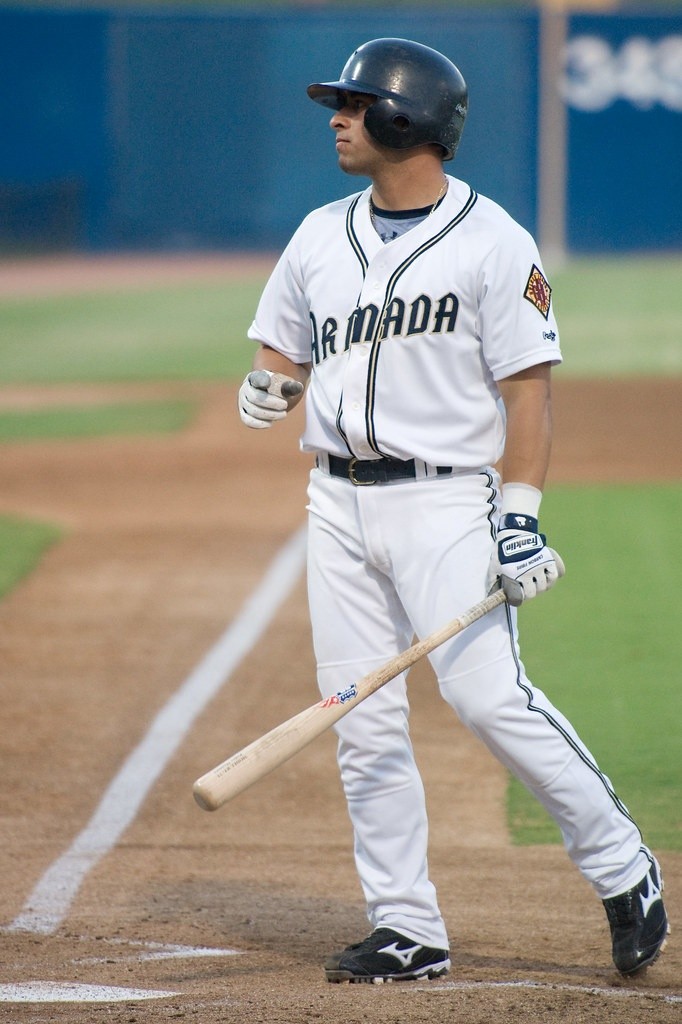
[486,513,558,607]
[239,370,304,428]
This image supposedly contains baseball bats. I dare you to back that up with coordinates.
[192,544,567,812]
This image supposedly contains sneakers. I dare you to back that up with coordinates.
[324,928,451,983]
[602,855,672,977]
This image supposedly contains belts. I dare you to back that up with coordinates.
[316,454,452,486]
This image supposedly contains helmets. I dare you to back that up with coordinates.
[307,38,468,161]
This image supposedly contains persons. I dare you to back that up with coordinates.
[238,37,671,984]
